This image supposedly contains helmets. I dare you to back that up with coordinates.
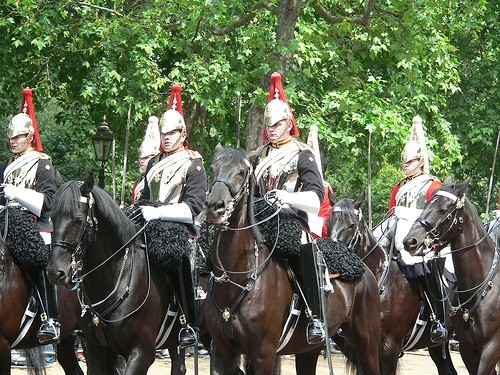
[307,125,325,187]
[158,85,188,151]
[262,72,299,141]
[138,116,160,175]
[400,114,429,174]
[7,87,43,151]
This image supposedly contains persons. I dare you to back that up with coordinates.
[250,72,330,344]
[132,84,206,345]
[306,125,337,240]
[0,87,60,340]
[389,115,447,343]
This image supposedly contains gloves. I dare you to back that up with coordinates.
[4,184,16,200]
[394,205,410,220]
[275,190,293,205]
[140,206,162,221]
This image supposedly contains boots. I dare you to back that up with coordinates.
[169,258,197,345]
[419,260,448,338]
[298,243,325,340]
[30,264,60,343]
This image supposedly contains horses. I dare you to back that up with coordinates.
[0,184,78,375]
[325,184,459,375]
[401,174,500,375]
[203,140,382,375]
[46,165,211,375]
